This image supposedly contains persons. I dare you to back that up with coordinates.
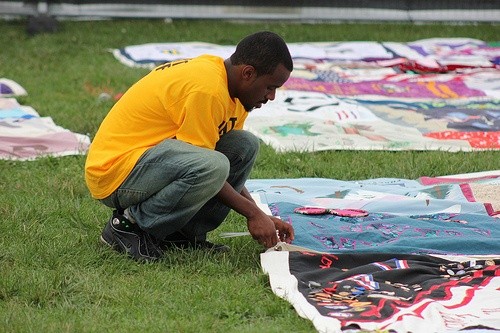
[85,31,294,263]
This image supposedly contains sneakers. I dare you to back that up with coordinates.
[166,232,230,256]
[207,242,208,244]
[100,211,163,265]
